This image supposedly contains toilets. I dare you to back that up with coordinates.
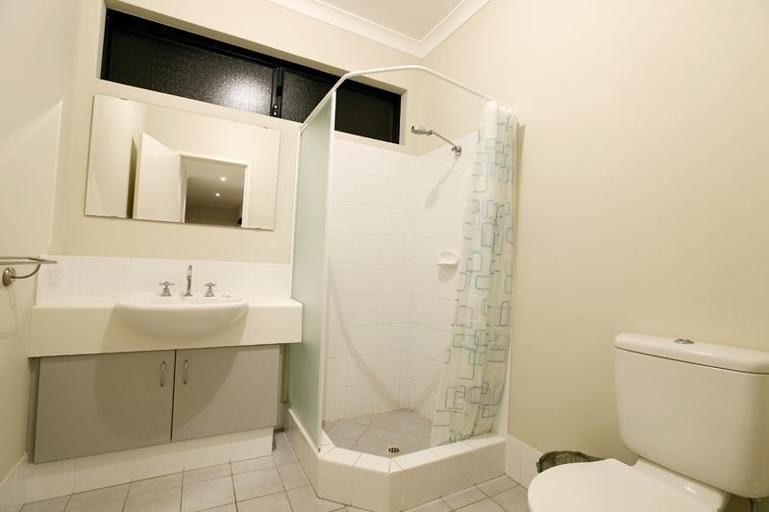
[527,331,769,511]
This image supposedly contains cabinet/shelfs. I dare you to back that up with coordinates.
[30,346,280,468]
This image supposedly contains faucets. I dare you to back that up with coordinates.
[183,262,194,296]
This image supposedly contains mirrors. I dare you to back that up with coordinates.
[83,93,282,232]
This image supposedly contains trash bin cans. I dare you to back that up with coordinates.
[536,450,605,473]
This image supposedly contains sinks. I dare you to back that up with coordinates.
[113,291,246,335]
